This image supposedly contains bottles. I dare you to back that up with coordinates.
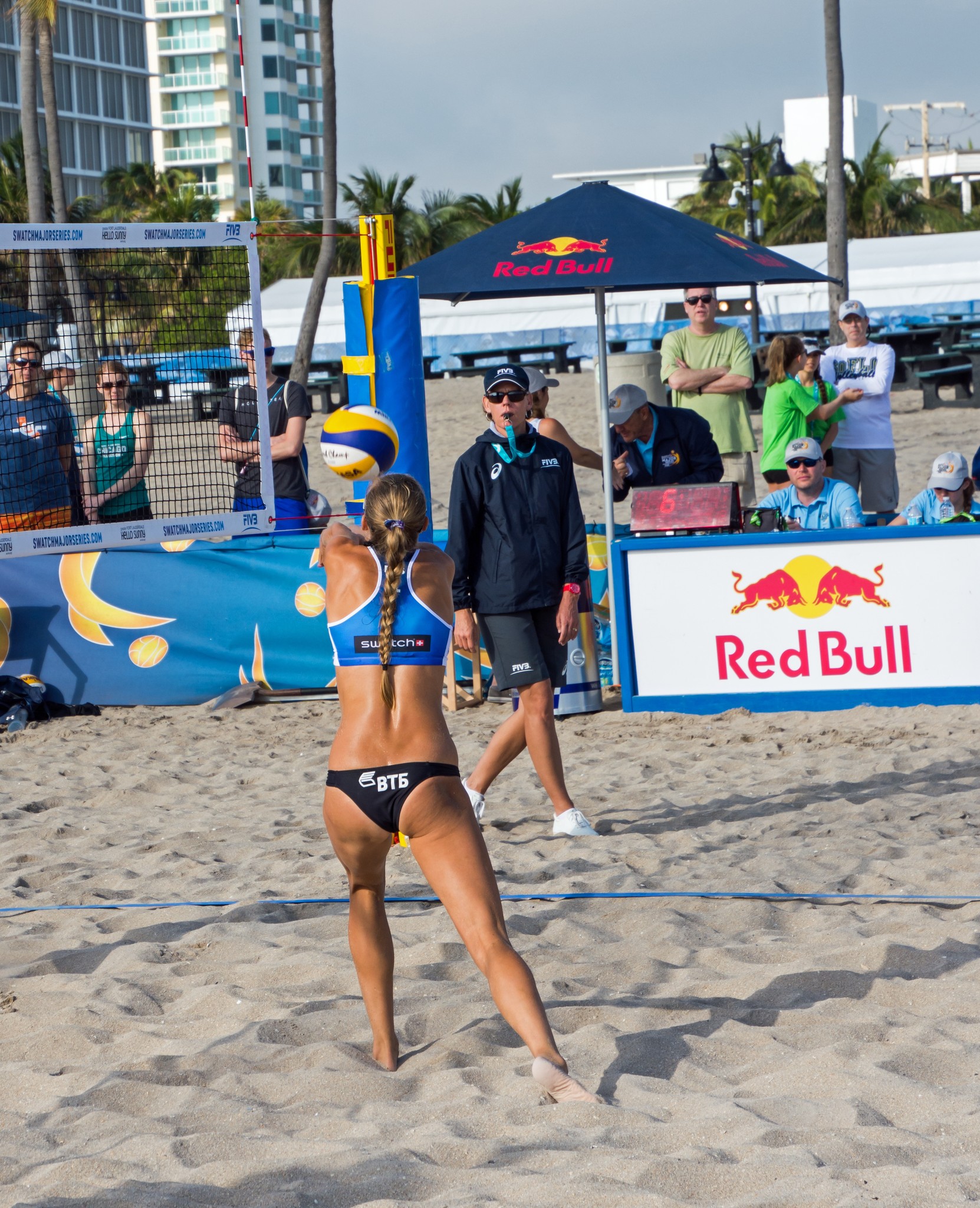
[907,503,923,526]
[939,497,954,523]
[8,709,29,733]
[842,507,858,527]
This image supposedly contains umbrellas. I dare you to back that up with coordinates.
[396,181,843,693]
[0,300,54,329]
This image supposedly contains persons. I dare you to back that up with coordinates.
[316,473,599,1104]
[42,351,90,527]
[0,340,76,534]
[520,288,980,534]
[215,327,311,537]
[443,363,598,837]
[81,359,153,525]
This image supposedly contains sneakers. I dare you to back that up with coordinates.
[553,808,599,836]
[462,777,485,830]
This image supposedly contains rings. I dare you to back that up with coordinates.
[572,628,577,631]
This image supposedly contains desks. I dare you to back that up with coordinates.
[903,321,980,346]
[866,328,944,383]
[931,312,980,321]
[126,364,165,403]
[450,341,577,380]
[423,356,442,378]
[759,328,830,352]
[199,359,346,420]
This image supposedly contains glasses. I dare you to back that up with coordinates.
[801,339,819,346]
[10,357,42,369]
[935,481,966,492]
[483,389,528,404]
[843,317,861,324]
[241,346,275,358]
[787,458,821,469]
[685,294,714,306]
[100,379,129,390]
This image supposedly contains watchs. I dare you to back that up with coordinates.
[563,583,580,594]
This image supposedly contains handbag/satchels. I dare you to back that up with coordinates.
[939,511,980,524]
[742,506,789,534]
[304,488,332,530]
[0,675,51,725]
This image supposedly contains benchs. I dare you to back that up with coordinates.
[131,378,178,405]
[425,355,588,380]
[747,332,980,413]
[595,337,663,353]
[182,377,341,424]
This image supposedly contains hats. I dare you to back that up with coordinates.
[521,366,560,393]
[927,451,969,491]
[839,300,867,321]
[41,351,83,369]
[801,336,826,356]
[785,436,823,463]
[608,384,647,425]
[484,363,530,393]
